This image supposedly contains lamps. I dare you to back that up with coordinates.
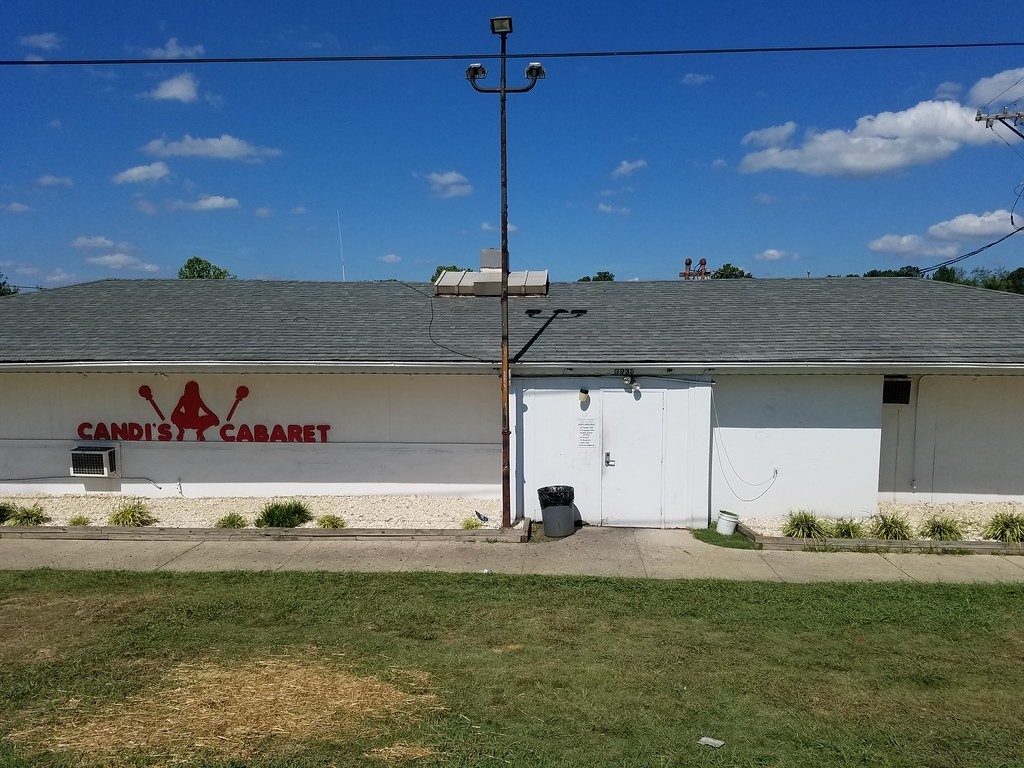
[622,374,641,390]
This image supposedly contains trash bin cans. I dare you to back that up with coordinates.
[537,485,575,538]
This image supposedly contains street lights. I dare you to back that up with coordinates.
[465,14,547,533]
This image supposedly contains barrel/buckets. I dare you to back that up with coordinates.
[716,509,739,535]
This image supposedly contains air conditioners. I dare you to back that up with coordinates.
[68,445,118,479]
